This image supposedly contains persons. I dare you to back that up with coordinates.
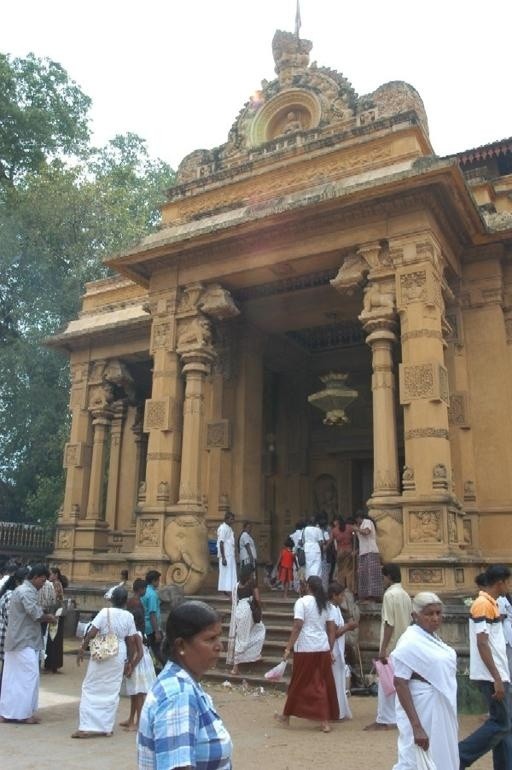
[282,111,303,134]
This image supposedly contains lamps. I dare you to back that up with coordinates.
[306,369,361,426]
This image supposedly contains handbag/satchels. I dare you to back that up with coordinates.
[297,548,305,566]
[87,632,119,663]
[248,598,261,625]
